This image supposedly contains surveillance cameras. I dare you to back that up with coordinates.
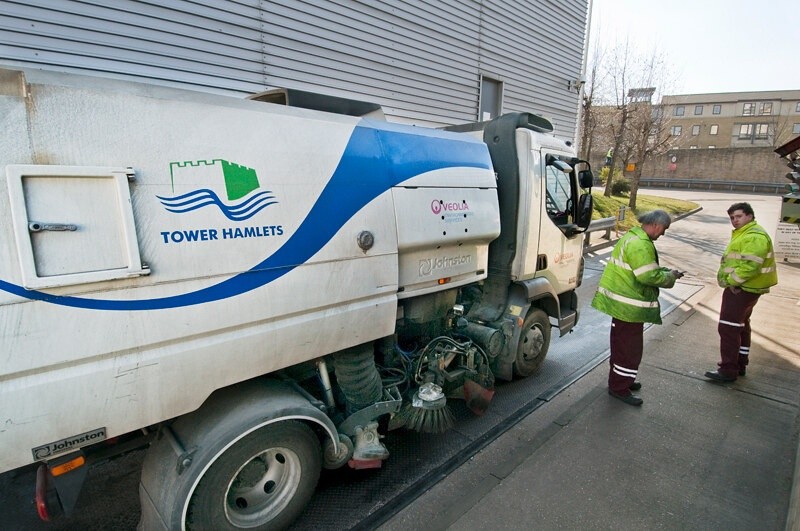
[577,78,586,85]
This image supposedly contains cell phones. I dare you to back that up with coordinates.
[680,271,686,274]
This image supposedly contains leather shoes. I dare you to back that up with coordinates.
[609,391,643,405]
[630,380,641,390]
[704,370,737,381]
[718,362,745,376]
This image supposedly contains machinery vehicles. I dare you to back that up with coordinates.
[0,62,594,531]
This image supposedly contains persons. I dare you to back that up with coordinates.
[591,209,683,406]
[605,147,614,165]
[704,204,777,380]
